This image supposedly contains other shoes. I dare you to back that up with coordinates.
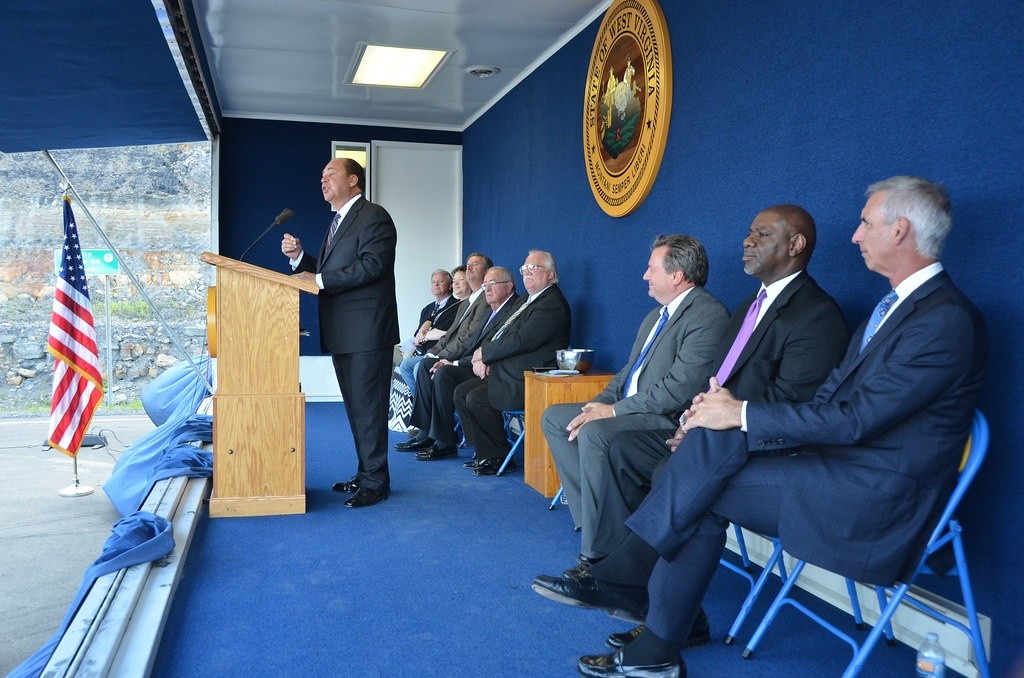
[407,430,419,437]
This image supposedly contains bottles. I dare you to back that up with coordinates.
[915,632,945,678]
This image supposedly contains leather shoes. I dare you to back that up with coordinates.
[472,457,516,475]
[561,556,606,580]
[576,554,594,564]
[414,444,458,461]
[604,613,710,650]
[394,436,434,452]
[333,478,361,493]
[462,457,495,469]
[531,573,649,623]
[577,645,686,678]
[344,484,388,507]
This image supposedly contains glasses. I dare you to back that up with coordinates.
[480,280,508,290]
[519,264,546,275]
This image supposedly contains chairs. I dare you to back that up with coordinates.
[454,346,571,477]
[548,405,990,678]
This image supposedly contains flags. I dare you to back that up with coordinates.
[45,196,103,459]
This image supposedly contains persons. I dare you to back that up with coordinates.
[453,250,572,477]
[540,233,734,580]
[531,174,987,678]
[591,204,850,652]
[400,265,473,438]
[281,158,400,508]
[393,266,521,461]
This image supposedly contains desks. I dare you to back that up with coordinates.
[523,370,617,499]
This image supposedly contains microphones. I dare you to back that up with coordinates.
[240,207,294,262]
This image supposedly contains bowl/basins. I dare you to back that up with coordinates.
[557,349,595,374]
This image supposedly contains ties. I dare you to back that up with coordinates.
[859,290,898,355]
[623,307,669,400]
[491,299,531,342]
[431,303,440,316]
[326,213,341,249]
[484,311,496,330]
[708,289,767,393]
